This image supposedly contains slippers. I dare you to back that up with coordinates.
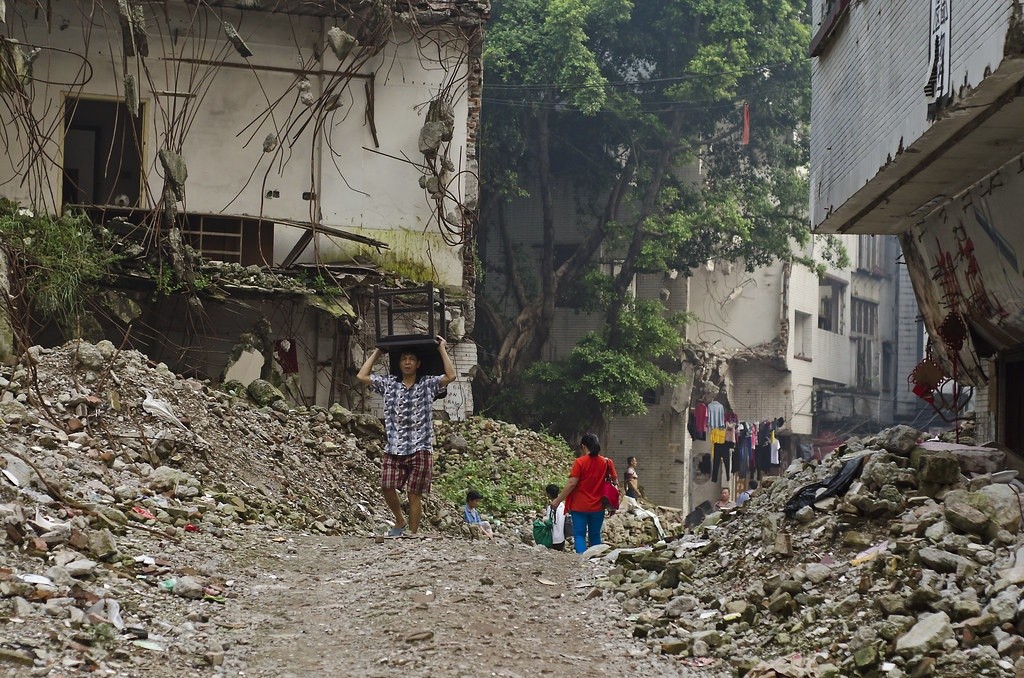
[390,523,408,536]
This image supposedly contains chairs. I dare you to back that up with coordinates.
[372,282,448,400]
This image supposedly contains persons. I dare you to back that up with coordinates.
[551,432,620,554]
[462,491,494,540]
[736,480,759,508]
[624,457,642,501]
[356,335,456,537]
[714,488,737,513]
[543,484,568,552]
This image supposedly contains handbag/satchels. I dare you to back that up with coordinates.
[602,458,619,509]
[534,507,556,548]
